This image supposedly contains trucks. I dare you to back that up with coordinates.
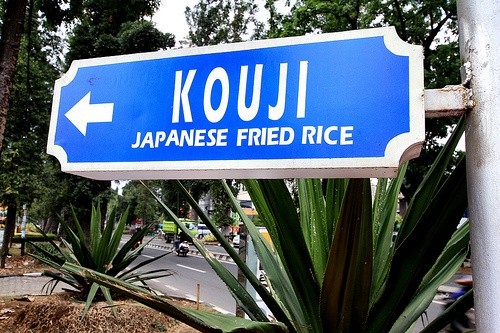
[162,218,199,245]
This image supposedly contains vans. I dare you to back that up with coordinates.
[198,223,212,239]
[232,227,269,248]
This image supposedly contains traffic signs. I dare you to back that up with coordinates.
[46,24,426,178]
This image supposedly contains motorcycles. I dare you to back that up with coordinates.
[174,235,190,256]
[132,238,143,255]
